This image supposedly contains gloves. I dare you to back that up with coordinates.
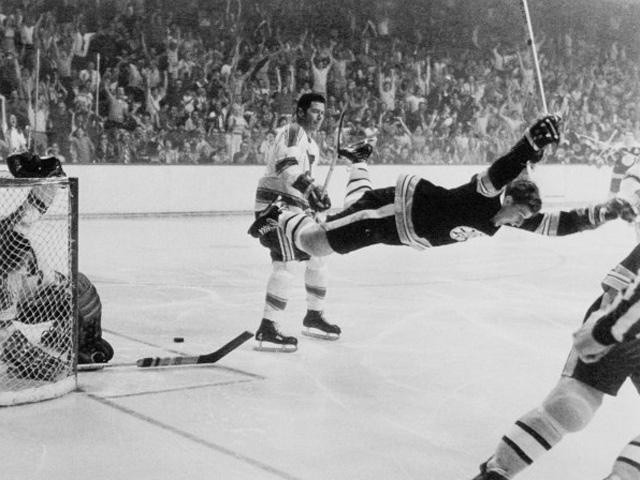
[593,196,637,227]
[307,186,332,212]
[6,152,62,178]
[528,114,562,149]
[1,330,67,382]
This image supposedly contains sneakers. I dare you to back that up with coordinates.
[250,205,284,238]
[302,309,340,334]
[256,318,297,345]
[338,143,372,163]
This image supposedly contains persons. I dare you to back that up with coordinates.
[0,167,115,385]
[470,243,640,480]
[590,136,638,194]
[1,0,639,165]
[0,91,69,189]
[247,116,638,262]
[254,90,343,345]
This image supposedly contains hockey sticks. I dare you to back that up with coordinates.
[70,330,255,373]
[317,110,346,199]
